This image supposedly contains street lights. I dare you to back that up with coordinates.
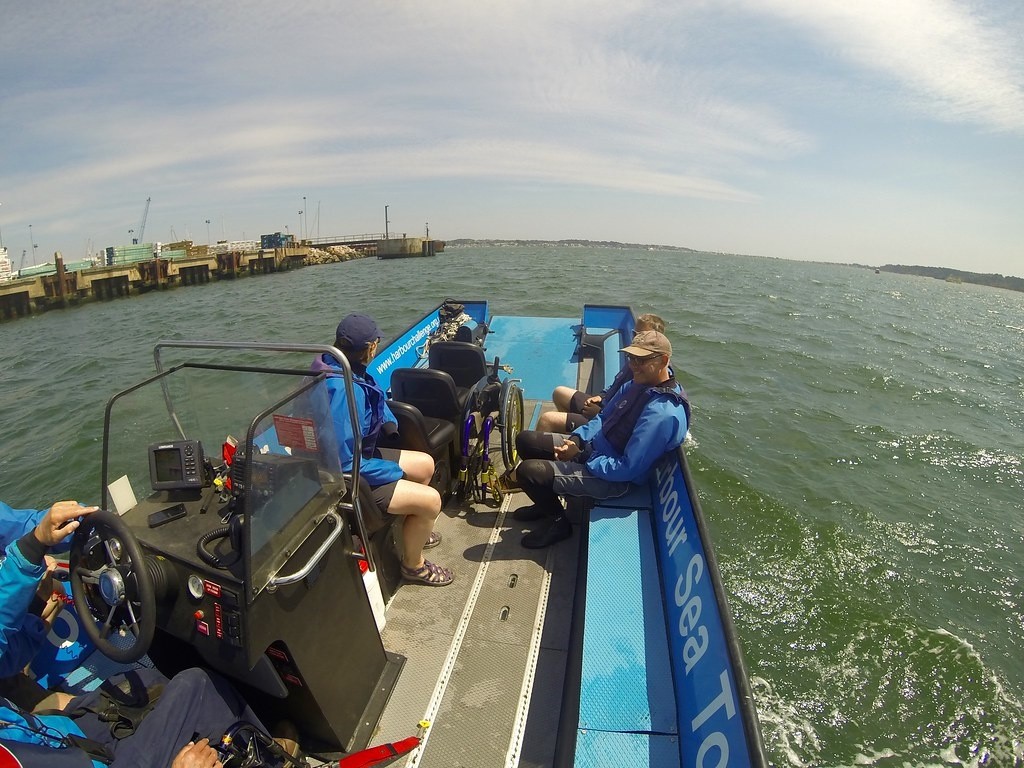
[206,220,210,246]
[303,196,307,239]
[385,205,389,240]
[29,224,38,266]
[298,210,303,240]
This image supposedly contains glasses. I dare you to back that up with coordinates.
[15,712,67,749]
[376,337,382,345]
[625,354,662,365]
[632,329,636,336]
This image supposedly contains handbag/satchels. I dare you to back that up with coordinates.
[72,678,166,740]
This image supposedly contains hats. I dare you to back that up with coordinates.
[620,331,673,357]
[336,312,386,352]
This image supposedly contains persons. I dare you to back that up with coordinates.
[486,314,666,493]
[514,329,692,548]
[290,313,455,587]
[0,499,305,767]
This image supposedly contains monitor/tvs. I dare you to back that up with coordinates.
[153,449,183,482]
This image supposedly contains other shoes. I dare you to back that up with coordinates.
[271,719,300,768]
[521,516,572,548]
[514,502,545,521]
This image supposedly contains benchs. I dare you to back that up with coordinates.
[572,335,681,768]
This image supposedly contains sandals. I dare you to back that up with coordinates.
[401,558,455,586]
[423,531,442,549]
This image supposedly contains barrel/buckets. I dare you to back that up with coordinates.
[357,558,386,634]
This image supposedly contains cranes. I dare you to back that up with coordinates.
[129,196,151,244]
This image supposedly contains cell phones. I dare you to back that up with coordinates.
[148,503,187,528]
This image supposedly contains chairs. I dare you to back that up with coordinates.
[381,400,453,452]
[343,474,398,537]
[390,370,473,423]
[427,341,487,389]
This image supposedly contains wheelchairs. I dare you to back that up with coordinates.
[454,357,525,506]
